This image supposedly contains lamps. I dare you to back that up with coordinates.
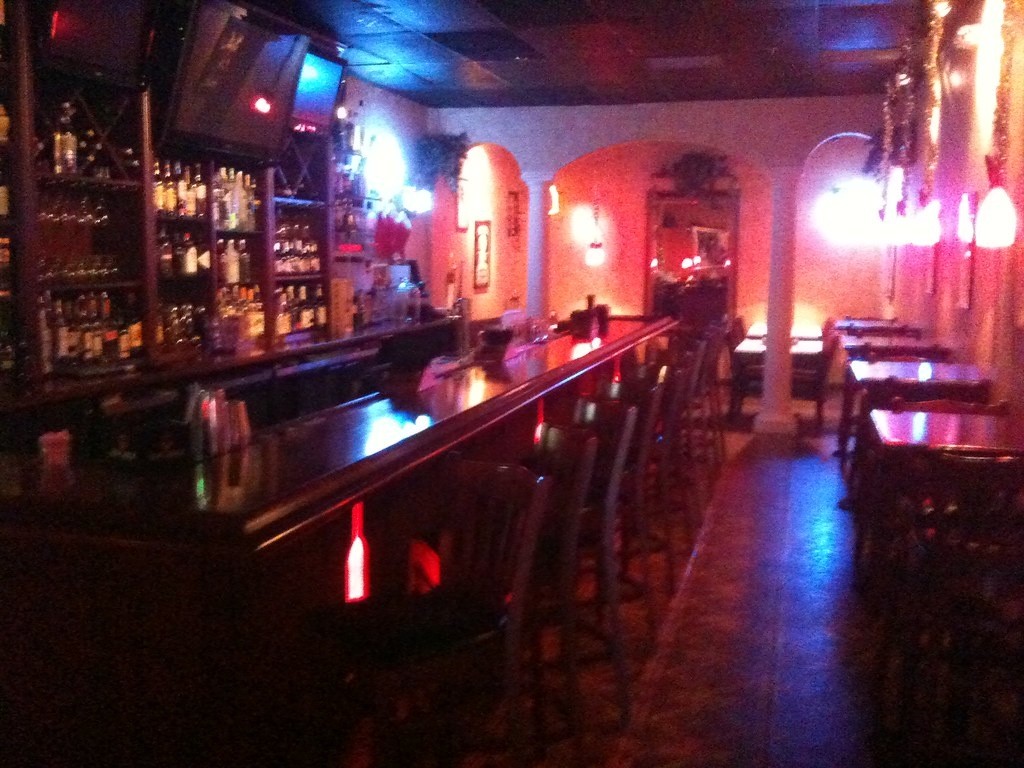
[585,181,606,267]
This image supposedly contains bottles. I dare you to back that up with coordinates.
[206,388,232,453]
[39,432,76,504]
[53,103,77,179]
[585,296,599,340]
[355,290,370,330]
[39,158,326,376]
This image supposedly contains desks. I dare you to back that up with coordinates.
[734,338,824,358]
[840,335,941,362]
[854,409,1024,601]
[746,320,822,339]
[851,358,989,411]
[841,314,920,335]
[0,320,688,768]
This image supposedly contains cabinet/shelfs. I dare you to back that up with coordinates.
[13,0,336,394]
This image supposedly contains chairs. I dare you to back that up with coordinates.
[354,318,747,768]
[792,318,838,432]
[726,316,763,421]
[840,314,1024,723]
